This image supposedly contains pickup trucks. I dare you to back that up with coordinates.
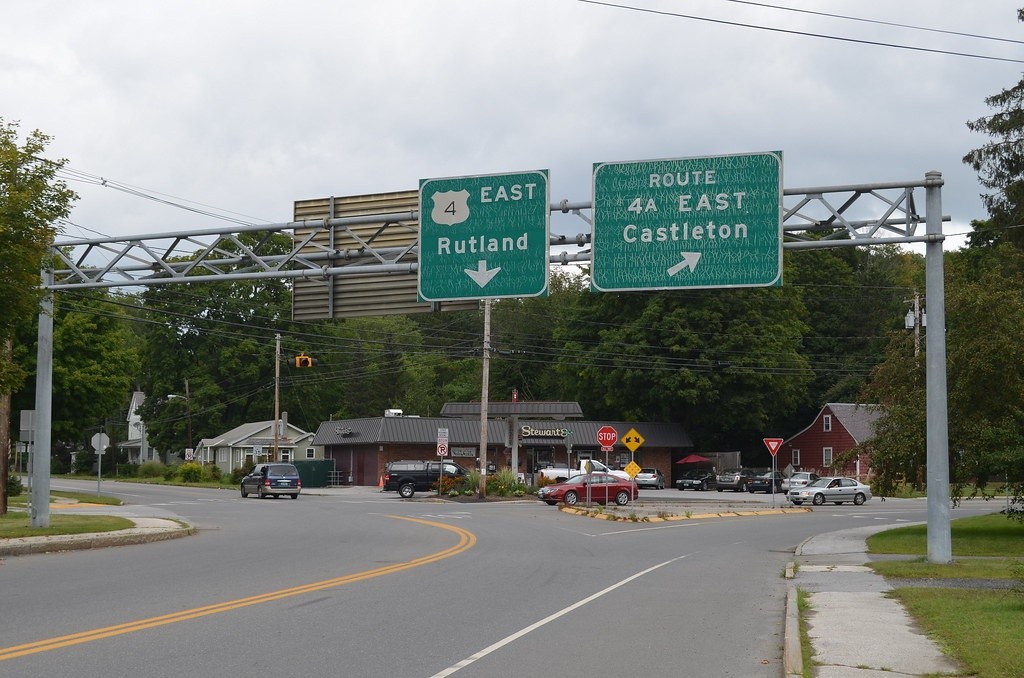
[539,459,631,491]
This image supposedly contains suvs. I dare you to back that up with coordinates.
[382,458,470,498]
[241,463,302,500]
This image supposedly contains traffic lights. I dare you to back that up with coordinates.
[310,355,319,368]
[287,356,296,368]
[296,356,311,368]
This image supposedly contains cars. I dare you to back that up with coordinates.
[634,467,665,490]
[781,471,819,495]
[716,467,755,492]
[537,472,639,507]
[676,469,717,491]
[786,476,873,505]
[744,468,788,494]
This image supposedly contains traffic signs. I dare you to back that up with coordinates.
[589,148,784,293]
[417,167,551,304]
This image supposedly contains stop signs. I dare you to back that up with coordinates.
[597,426,617,448]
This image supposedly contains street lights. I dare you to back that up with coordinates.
[168,394,192,463]
